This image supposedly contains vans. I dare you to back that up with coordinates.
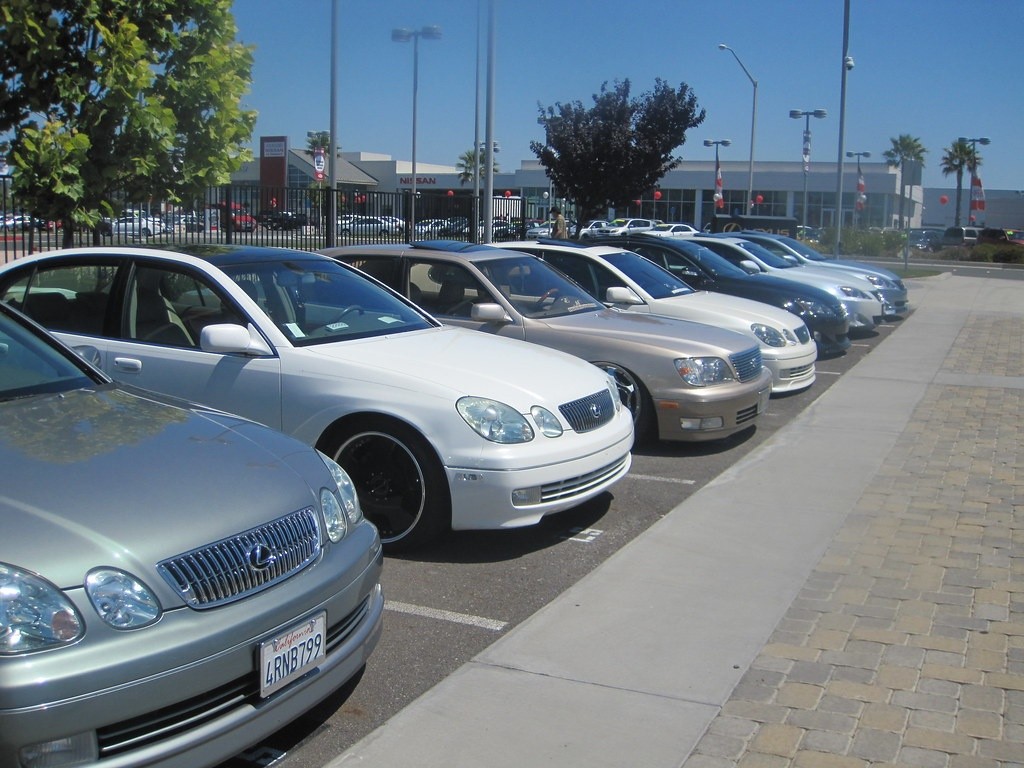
[212,202,258,233]
[710,214,832,244]
[944,225,988,246]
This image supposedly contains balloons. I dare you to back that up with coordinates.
[969,216,976,222]
[654,191,661,199]
[542,192,549,199]
[940,195,948,204]
[713,193,720,201]
[859,194,867,203]
[636,200,640,205]
[756,195,763,202]
[504,191,511,197]
[448,190,453,197]
[354,189,365,203]
[269,198,277,207]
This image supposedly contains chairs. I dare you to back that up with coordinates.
[22,280,259,351]
[429,262,473,320]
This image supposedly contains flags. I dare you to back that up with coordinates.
[855,169,865,212]
[970,170,986,210]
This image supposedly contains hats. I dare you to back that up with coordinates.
[549,206,558,213]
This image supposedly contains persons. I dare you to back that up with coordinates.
[549,207,566,239]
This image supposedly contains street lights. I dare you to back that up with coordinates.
[703,139,733,214]
[787,108,829,235]
[845,151,873,231]
[718,44,761,215]
[954,137,1001,233]
[387,25,444,239]
[835,54,856,258]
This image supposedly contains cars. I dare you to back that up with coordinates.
[0,301,385,768]
[596,218,659,240]
[609,237,853,356]
[0,242,634,552]
[567,219,610,239]
[906,227,948,255]
[976,227,1024,248]
[701,237,888,335]
[631,224,699,241]
[694,231,912,314]
[75,208,222,238]
[174,241,774,455]
[526,220,575,240]
[406,238,821,391]
[0,213,65,234]
[323,214,521,240]
[249,208,308,231]
